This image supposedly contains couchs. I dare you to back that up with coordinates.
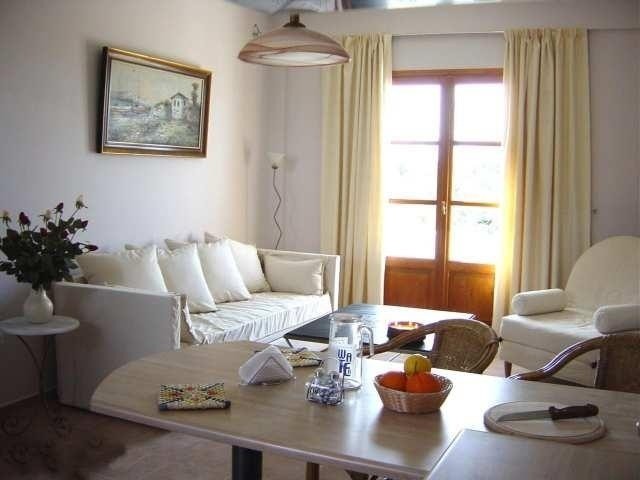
[43,237,348,434]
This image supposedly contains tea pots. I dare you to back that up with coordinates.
[327,313,374,392]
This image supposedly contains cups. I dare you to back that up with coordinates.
[306,357,347,405]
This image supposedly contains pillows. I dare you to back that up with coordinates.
[98,280,197,343]
[77,244,171,304]
[162,237,250,304]
[225,238,272,296]
[261,251,325,298]
[129,240,218,316]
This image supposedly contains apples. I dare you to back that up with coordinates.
[404,353,432,376]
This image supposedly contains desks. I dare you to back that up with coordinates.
[88,336,639,480]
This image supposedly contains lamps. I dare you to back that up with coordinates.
[266,151,286,252]
[237,11,351,69]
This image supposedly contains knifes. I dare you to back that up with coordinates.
[498,402,599,423]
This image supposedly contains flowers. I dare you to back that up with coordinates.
[0,193,97,288]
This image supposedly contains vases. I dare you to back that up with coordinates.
[21,284,52,321]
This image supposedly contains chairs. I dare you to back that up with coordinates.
[509,333,640,398]
[500,234,640,376]
[307,320,504,480]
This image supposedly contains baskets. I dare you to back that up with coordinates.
[373,372,453,413]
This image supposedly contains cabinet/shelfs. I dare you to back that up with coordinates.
[0,314,82,440]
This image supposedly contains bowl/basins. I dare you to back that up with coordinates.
[387,321,426,342]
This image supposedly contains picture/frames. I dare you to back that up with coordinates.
[95,43,213,160]
